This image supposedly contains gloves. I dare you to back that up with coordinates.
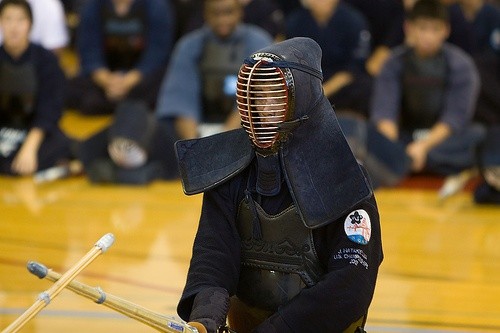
[185,318,218,333]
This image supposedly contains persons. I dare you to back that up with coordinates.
[275,0,500,121]
[0,0,88,176]
[176,36,384,333]
[71,1,176,116]
[0,0,70,63]
[369,0,481,206]
[157,0,274,142]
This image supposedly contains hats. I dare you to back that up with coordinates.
[173,37,373,230]
